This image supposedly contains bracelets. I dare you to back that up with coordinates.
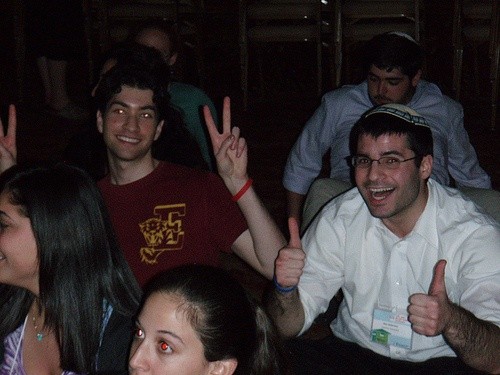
[273,274,296,292]
[231,178,253,202]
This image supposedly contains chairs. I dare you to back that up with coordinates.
[79,0,500,130]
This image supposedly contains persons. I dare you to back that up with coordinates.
[0,159,146,375]
[127,264,297,375]
[0,14,288,301]
[281,28,499,241]
[266,102,500,375]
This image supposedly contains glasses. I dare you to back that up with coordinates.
[350,152,419,170]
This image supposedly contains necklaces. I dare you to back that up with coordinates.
[32,314,43,340]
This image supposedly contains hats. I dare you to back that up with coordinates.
[363,103,431,128]
